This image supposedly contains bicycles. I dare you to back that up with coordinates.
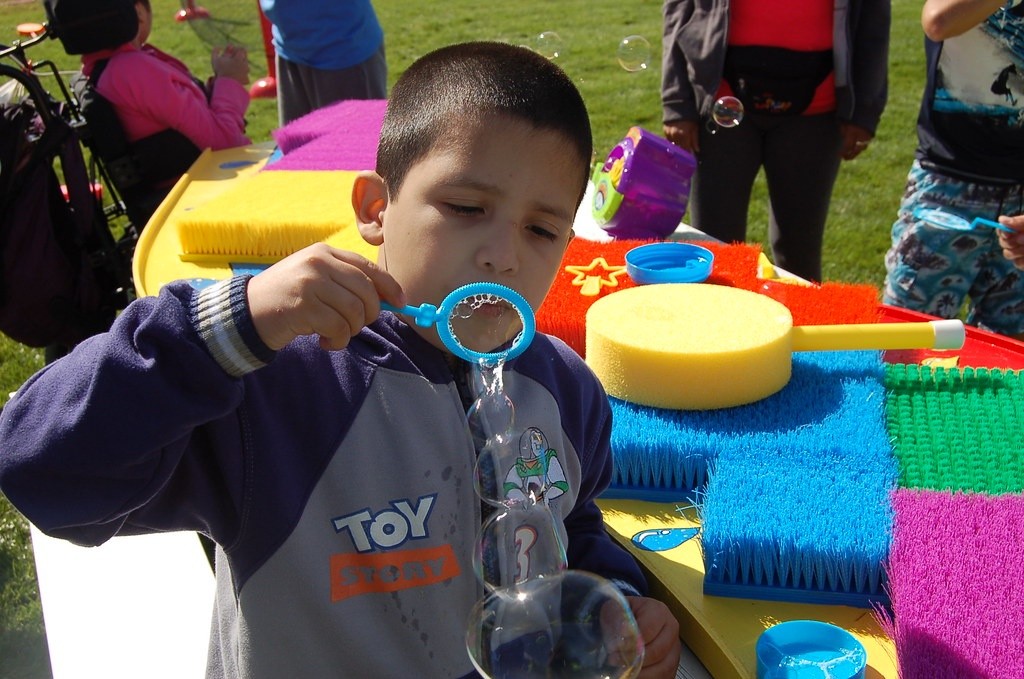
[1,20,140,322]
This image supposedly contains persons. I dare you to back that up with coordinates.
[1,40,684,679]
[883,0,1024,342]
[42,0,251,237]
[258,0,387,129]
[996,214,1024,270]
[661,0,891,287]
